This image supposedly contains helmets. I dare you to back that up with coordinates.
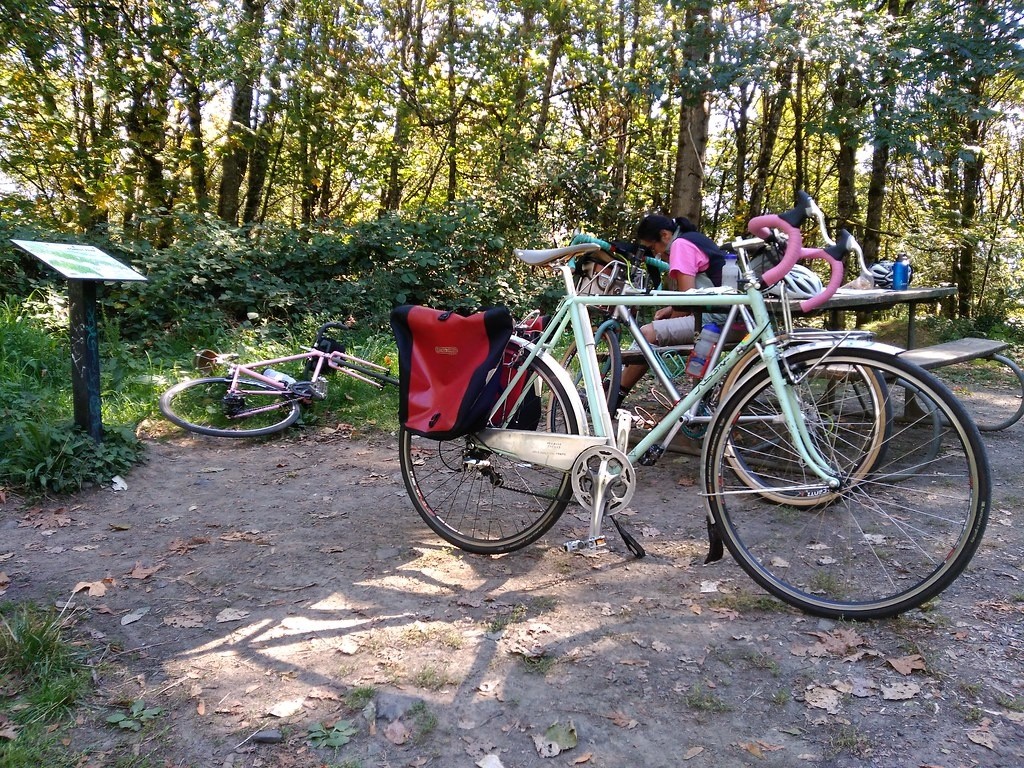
[871,258,914,287]
[766,264,824,300]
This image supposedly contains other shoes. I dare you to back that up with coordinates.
[578,387,591,413]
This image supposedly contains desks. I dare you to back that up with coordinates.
[615,285,1024,496]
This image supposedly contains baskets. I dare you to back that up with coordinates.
[572,256,648,318]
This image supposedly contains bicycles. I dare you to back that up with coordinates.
[397,190,993,622]
[158,321,400,438]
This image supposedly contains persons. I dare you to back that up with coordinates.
[578,216,739,415]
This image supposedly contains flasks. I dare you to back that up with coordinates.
[893,253,909,291]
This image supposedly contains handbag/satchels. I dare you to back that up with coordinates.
[388,302,514,443]
[483,314,560,432]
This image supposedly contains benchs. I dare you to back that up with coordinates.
[596,342,739,363]
[809,335,1014,380]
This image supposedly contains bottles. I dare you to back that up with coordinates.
[722,253,739,293]
[686,320,720,377]
[261,367,296,387]
